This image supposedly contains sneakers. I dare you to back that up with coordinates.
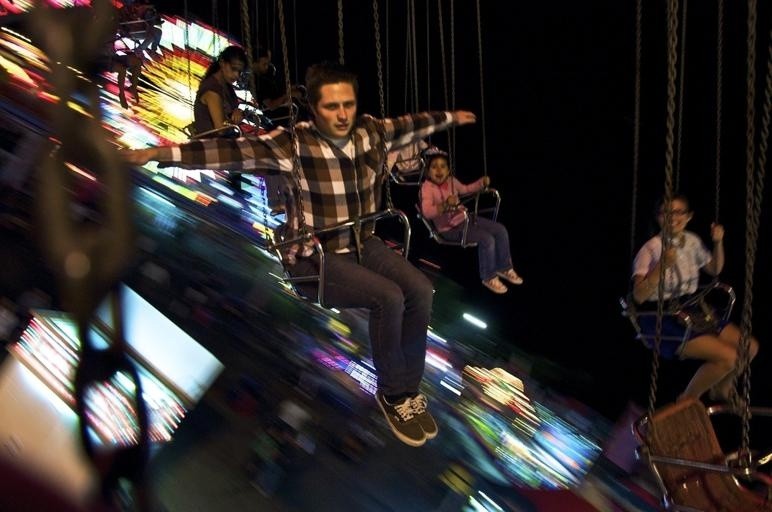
[406,394,439,440]
[375,386,427,446]
[496,267,523,284]
[481,276,507,294]
[709,390,754,420]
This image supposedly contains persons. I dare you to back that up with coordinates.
[109,63,476,449]
[247,46,303,125]
[393,139,449,183]
[628,187,760,419]
[415,150,524,296]
[193,46,250,135]
